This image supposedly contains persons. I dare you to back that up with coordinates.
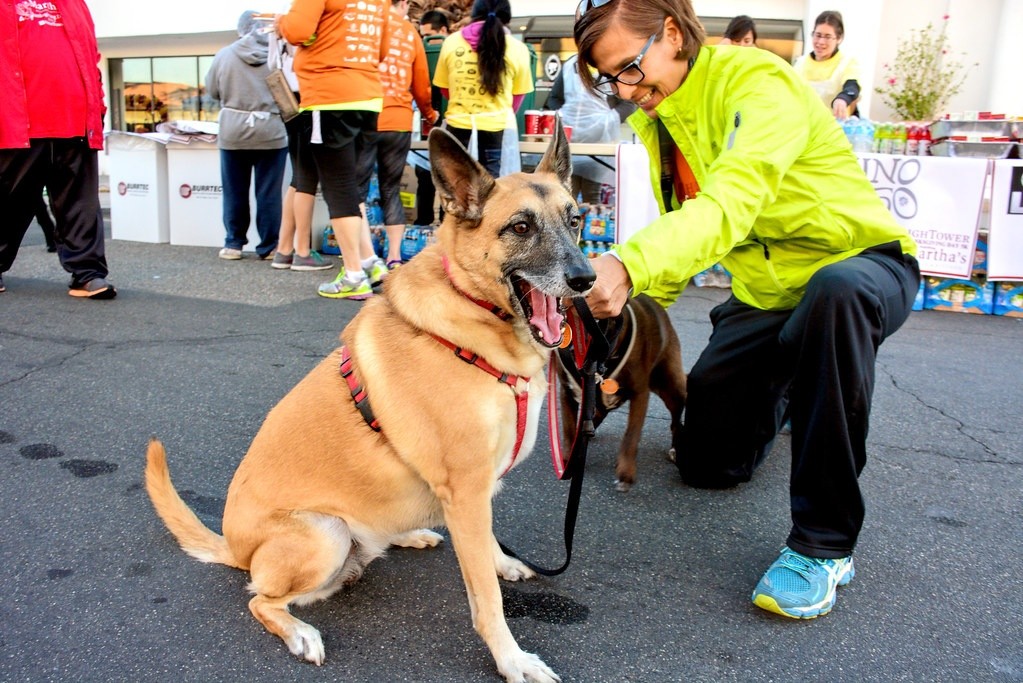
[571,0,921,620]
[0,0,861,301]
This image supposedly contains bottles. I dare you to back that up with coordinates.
[322,191,617,254]
[912,229,1022,312]
[834,117,932,155]
[694,262,732,289]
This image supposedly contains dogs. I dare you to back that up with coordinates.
[147,116,599,683]
[552,279,684,487]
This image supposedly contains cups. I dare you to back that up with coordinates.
[541,112,558,136]
[563,126,572,139]
[523,110,540,135]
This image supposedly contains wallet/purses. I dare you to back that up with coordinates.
[264,39,300,123]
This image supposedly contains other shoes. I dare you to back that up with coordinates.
[219,247,242,260]
[265,244,278,259]
[386,259,403,270]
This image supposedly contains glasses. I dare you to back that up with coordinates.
[574,0,611,23]
[592,33,655,97]
[812,32,837,41]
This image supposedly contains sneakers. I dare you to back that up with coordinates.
[318,258,389,299]
[271,249,334,270]
[750,545,856,620]
[68,277,117,299]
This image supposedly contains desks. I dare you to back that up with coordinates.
[406,137,620,175]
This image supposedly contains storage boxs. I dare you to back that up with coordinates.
[905,237,1023,317]
[321,209,614,262]
